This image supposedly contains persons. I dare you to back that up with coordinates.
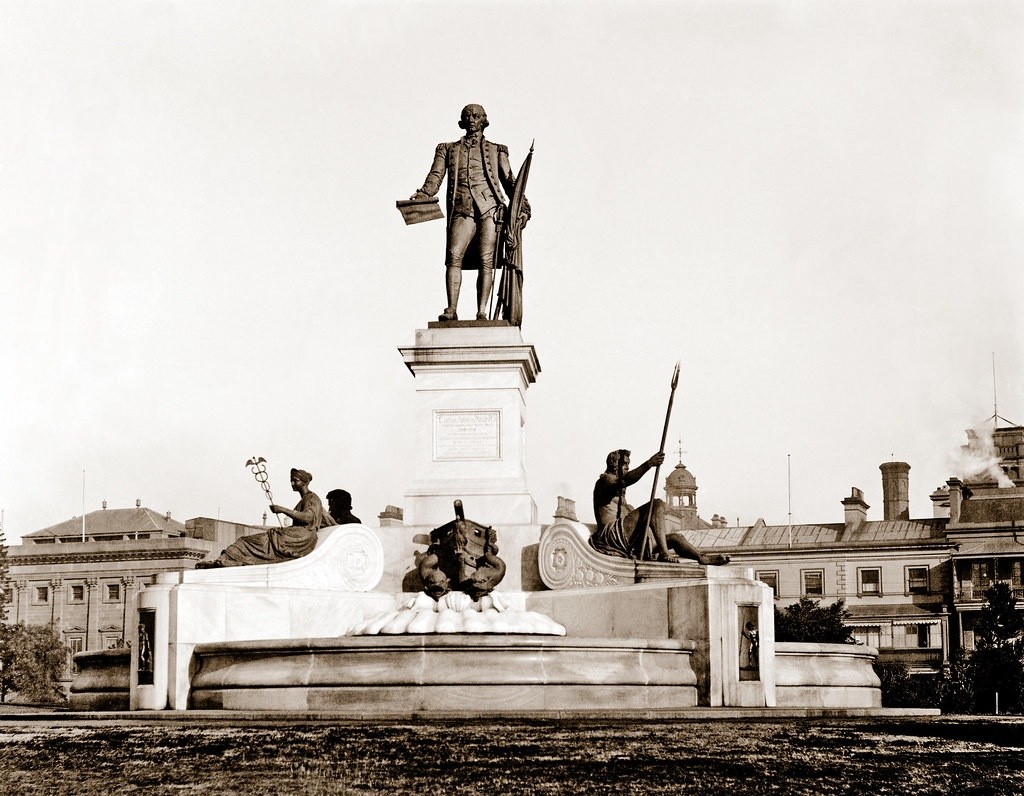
[593,449,731,566]
[138,624,151,670]
[410,103,531,321]
[743,621,759,669]
[324,488,361,528]
[194,467,323,569]
